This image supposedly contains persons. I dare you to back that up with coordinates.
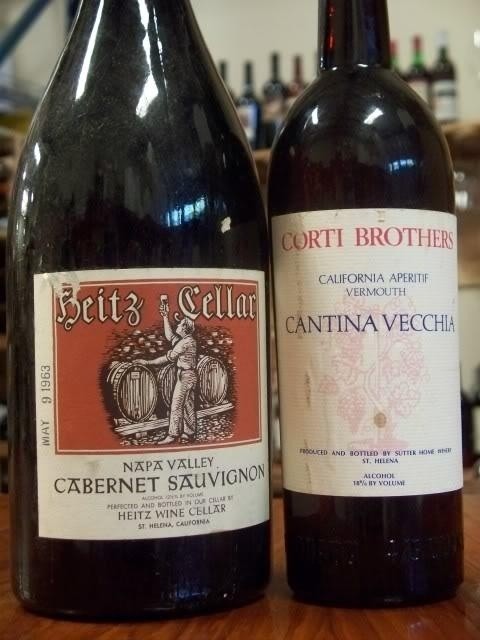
[132,302,199,444]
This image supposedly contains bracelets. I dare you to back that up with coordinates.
[145,360,149,366]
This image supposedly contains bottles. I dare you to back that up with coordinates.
[260,0,470,608]
[5,0,278,621]
